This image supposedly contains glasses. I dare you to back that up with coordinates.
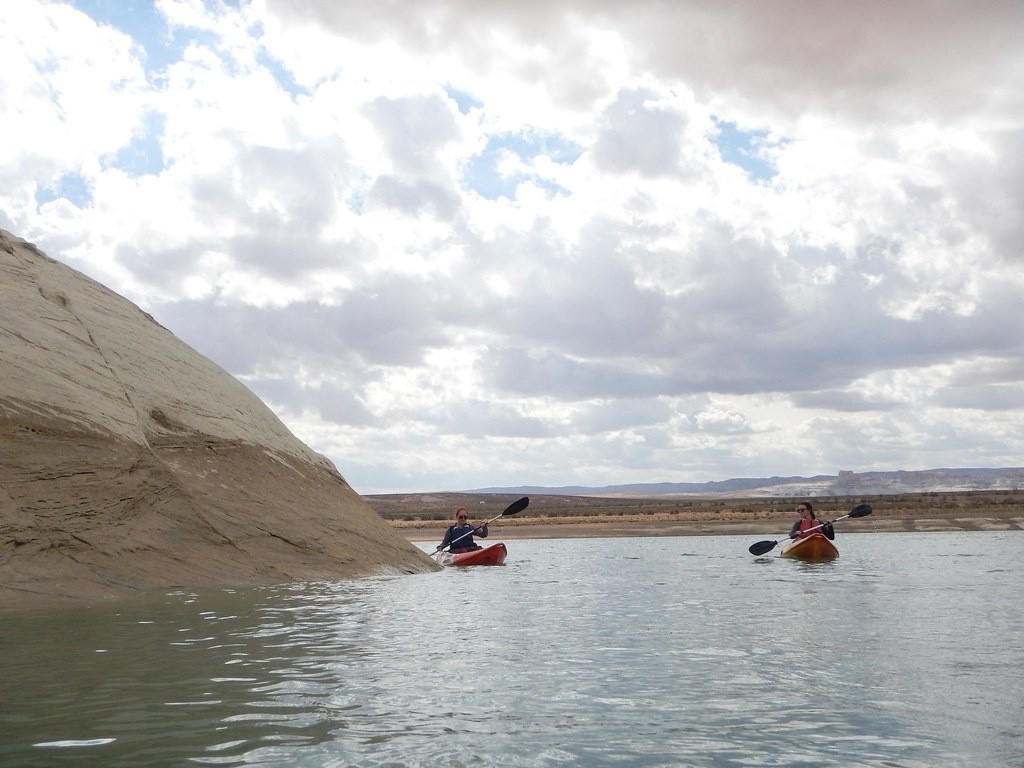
[795,508,809,513]
[459,515,468,519]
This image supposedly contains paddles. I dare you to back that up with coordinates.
[430,497,530,557]
[748,504,873,556]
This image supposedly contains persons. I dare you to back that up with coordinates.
[437,508,489,553]
[789,502,835,543]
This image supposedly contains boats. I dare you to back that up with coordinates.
[438,542,508,568]
[780,532,840,562]
